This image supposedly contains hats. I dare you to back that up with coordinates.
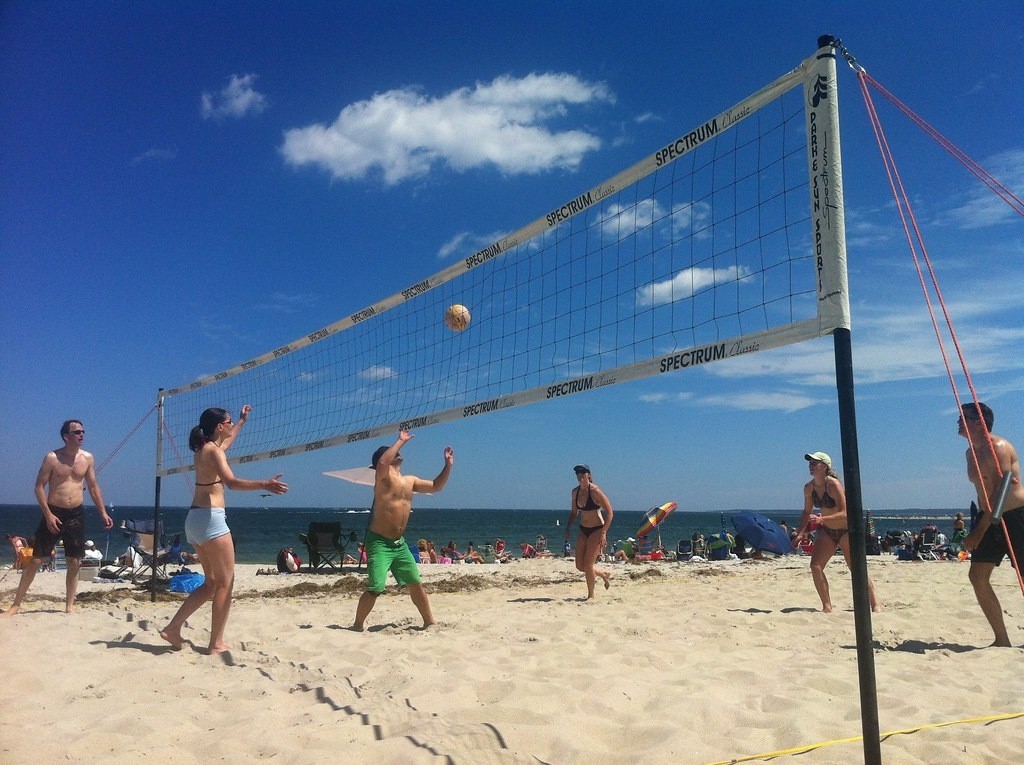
[574,463,590,472]
[626,537,636,541]
[805,452,831,467]
[85,540,94,547]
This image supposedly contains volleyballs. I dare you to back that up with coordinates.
[444,304,472,332]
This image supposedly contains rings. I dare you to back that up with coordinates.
[51,527,54,529]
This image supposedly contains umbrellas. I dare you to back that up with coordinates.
[322,463,434,568]
[731,510,793,555]
[636,502,677,548]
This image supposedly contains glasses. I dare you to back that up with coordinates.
[221,417,232,424]
[959,415,980,420]
[576,468,589,474]
[68,430,85,434]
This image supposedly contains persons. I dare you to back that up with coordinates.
[790,452,881,613]
[159,405,288,655]
[353,427,454,627]
[332,542,366,564]
[171,537,182,557]
[0,420,113,615]
[417,534,547,564]
[6,534,28,573]
[564,539,571,557]
[83,540,103,560]
[957,403,1024,647]
[611,532,746,563]
[43,545,55,562]
[564,464,613,602]
[779,512,967,555]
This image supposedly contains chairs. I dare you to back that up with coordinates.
[114,519,511,587]
[676,528,966,563]
